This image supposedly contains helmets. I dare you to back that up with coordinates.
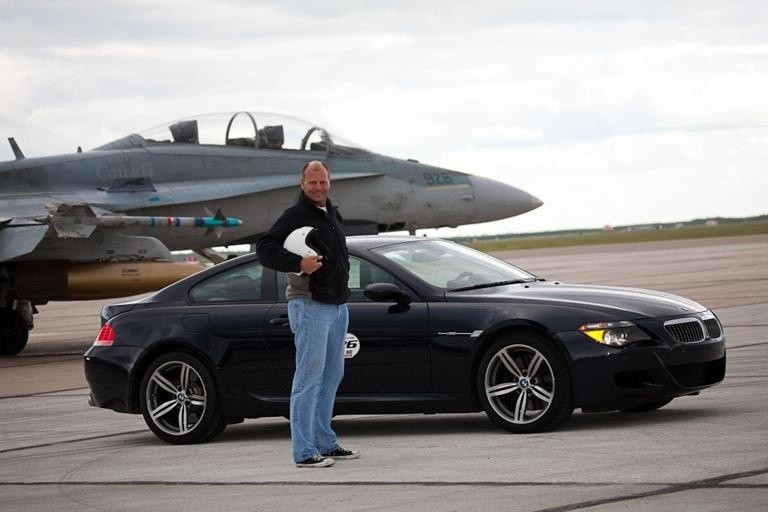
[283,226,328,276]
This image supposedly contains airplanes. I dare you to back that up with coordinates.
[0,109,545,357]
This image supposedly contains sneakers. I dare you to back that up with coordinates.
[321,446,359,459]
[295,452,334,467]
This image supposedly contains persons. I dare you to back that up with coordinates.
[255,158,361,470]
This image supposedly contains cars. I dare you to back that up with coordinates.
[82,235,730,446]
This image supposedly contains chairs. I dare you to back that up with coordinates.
[227,278,259,300]
